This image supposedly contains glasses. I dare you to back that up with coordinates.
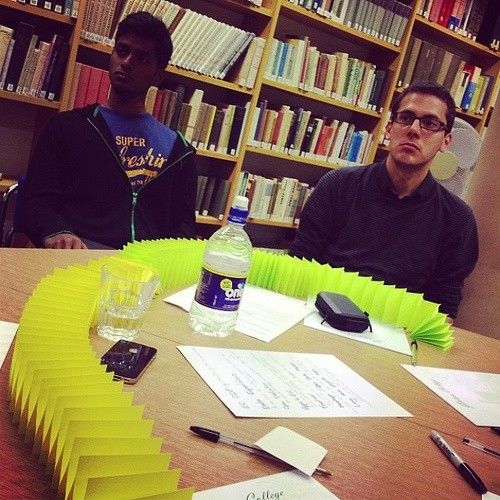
[392,112,449,132]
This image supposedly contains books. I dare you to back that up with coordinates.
[417,0,500,52]
[194,174,215,220]
[264,33,395,113]
[63,62,112,110]
[246,100,374,168]
[80,0,256,92]
[237,170,280,225]
[224,35,265,90]
[208,178,231,220]
[396,31,461,114]
[448,61,495,115]
[13,0,80,19]
[274,175,314,226]
[177,87,252,156]
[288,0,411,47]
[144,84,184,132]
[378,108,393,146]
[0,17,70,102]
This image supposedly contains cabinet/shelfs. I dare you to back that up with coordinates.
[0,0,500,247]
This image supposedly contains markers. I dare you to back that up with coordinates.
[430,430,487,495]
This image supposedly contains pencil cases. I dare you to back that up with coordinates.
[315,291,370,332]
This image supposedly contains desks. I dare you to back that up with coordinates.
[0,249,500,500]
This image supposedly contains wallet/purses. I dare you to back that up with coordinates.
[316,291,374,334]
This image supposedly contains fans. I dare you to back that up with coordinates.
[429,117,482,199]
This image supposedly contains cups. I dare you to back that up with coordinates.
[97,262,159,342]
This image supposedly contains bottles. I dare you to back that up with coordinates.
[187,195,254,337]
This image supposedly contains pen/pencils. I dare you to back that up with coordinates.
[462,436,500,458]
[411,339,417,366]
[190,426,334,477]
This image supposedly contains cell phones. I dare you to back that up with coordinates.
[99,339,157,386]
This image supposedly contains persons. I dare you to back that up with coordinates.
[19,12,198,249]
[290,80,478,321]
